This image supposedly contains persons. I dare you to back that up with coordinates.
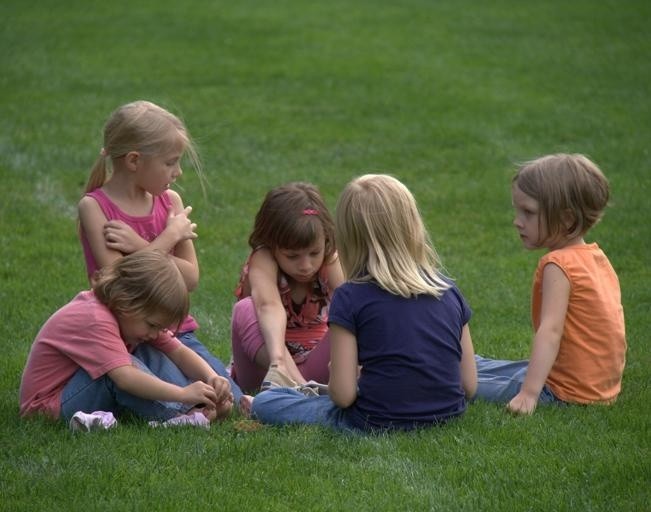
[239,173,478,441]
[77,101,245,416]
[462,152,627,420]
[229,183,345,393]
[18,249,234,424]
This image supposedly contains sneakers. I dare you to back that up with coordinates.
[162,412,211,432]
[239,394,256,420]
[261,365,329,397]
[69,410,119,432]
